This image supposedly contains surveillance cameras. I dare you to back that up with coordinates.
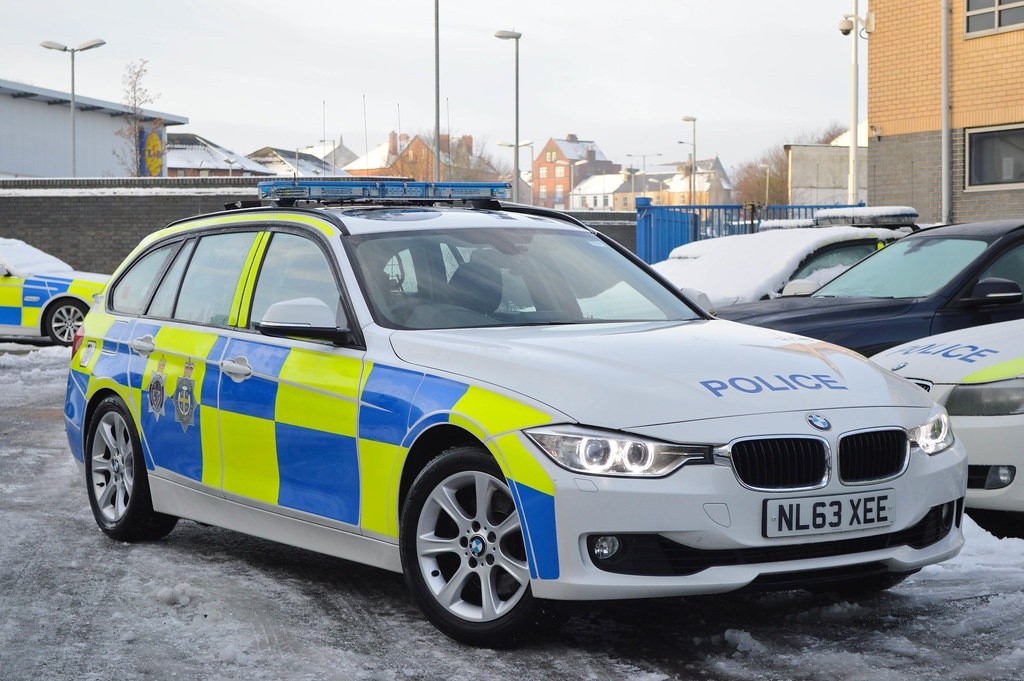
[838,11,874,35]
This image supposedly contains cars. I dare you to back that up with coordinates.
[702,221,1024,356]
[62,178,972,646]
[0,238,111,350]
[517,206,923,336]
[875,313,1023,534]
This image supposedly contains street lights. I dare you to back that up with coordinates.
[320,98,329,177]
[632,150,661,197]
[680,116,702,202]
[38,36,106,175]
[499,27,528,202]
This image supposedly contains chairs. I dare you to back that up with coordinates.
[443,258,504,315]
